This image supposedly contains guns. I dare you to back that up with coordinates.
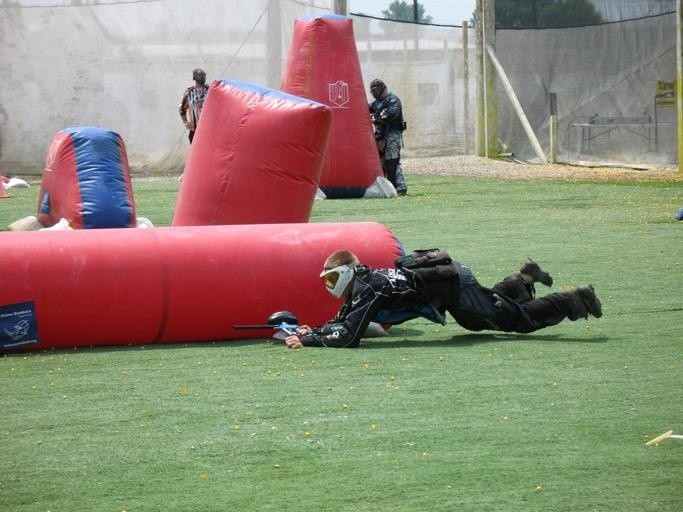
[234,324,347,339]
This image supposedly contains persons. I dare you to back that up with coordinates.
[179,68,210,142]
[284,248,603,348]
[368,78,409,195]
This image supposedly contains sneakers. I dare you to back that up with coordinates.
[578,283,602,318]
[398,189,407,195]
[520,258,553,287]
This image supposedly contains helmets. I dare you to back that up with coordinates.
[193,69,206,83]
[371,79,385,98]
